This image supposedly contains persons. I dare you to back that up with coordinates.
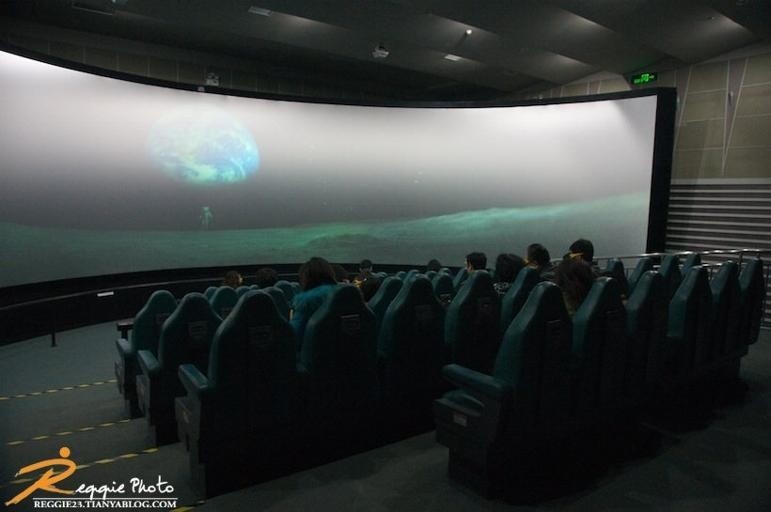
[256,267,277,288]
[299,257,349,289]
[526,239,595,304]
[494,254,524,296]
[225,270,243,289]
[465,252,487,274]
[354,259,376,287]
[428,260,441,271]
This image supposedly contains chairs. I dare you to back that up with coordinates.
[115,254,764,502]
